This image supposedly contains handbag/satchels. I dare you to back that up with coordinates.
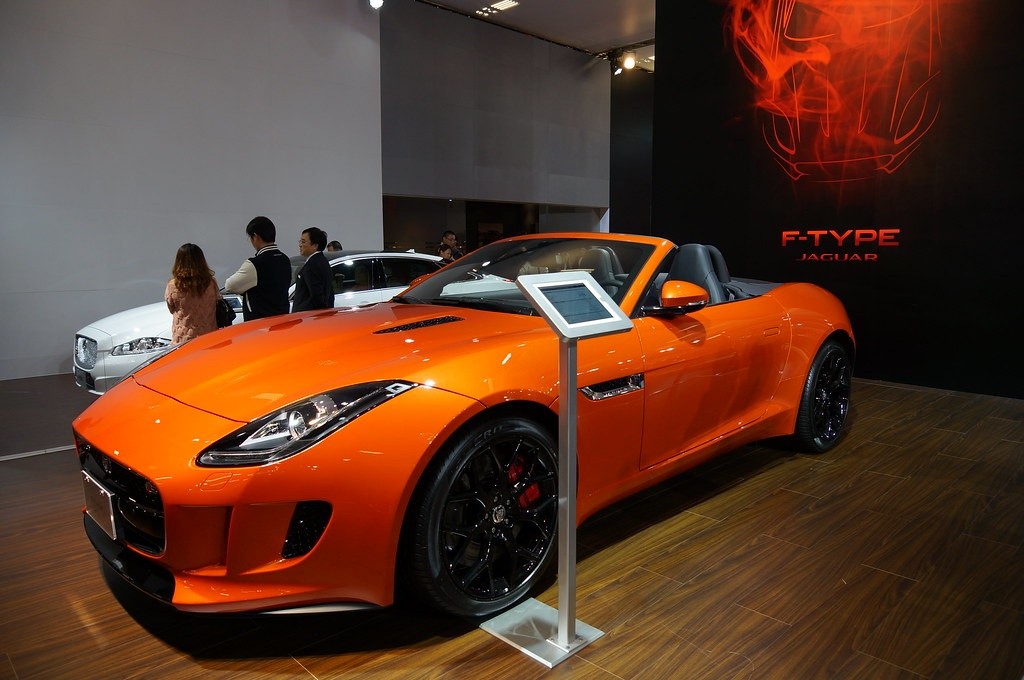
[211,277,236,327]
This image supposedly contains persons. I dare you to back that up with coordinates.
[166,243,224,343]
[435,231,589,280]
[291,228,335,313]
[327,241,343,251]
[225,216,292,322]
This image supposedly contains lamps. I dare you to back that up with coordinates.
[610,61,623,76]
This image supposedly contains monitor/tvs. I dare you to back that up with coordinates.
[541,284,614,324]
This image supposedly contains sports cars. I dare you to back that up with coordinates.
[69,230,856,621]
[70,249,527,402]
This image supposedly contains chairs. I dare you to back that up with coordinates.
[579,248,619,298]
[657,244,727,307]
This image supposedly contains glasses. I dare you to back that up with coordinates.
[445,238,457,242]
[298,240,313,245]
